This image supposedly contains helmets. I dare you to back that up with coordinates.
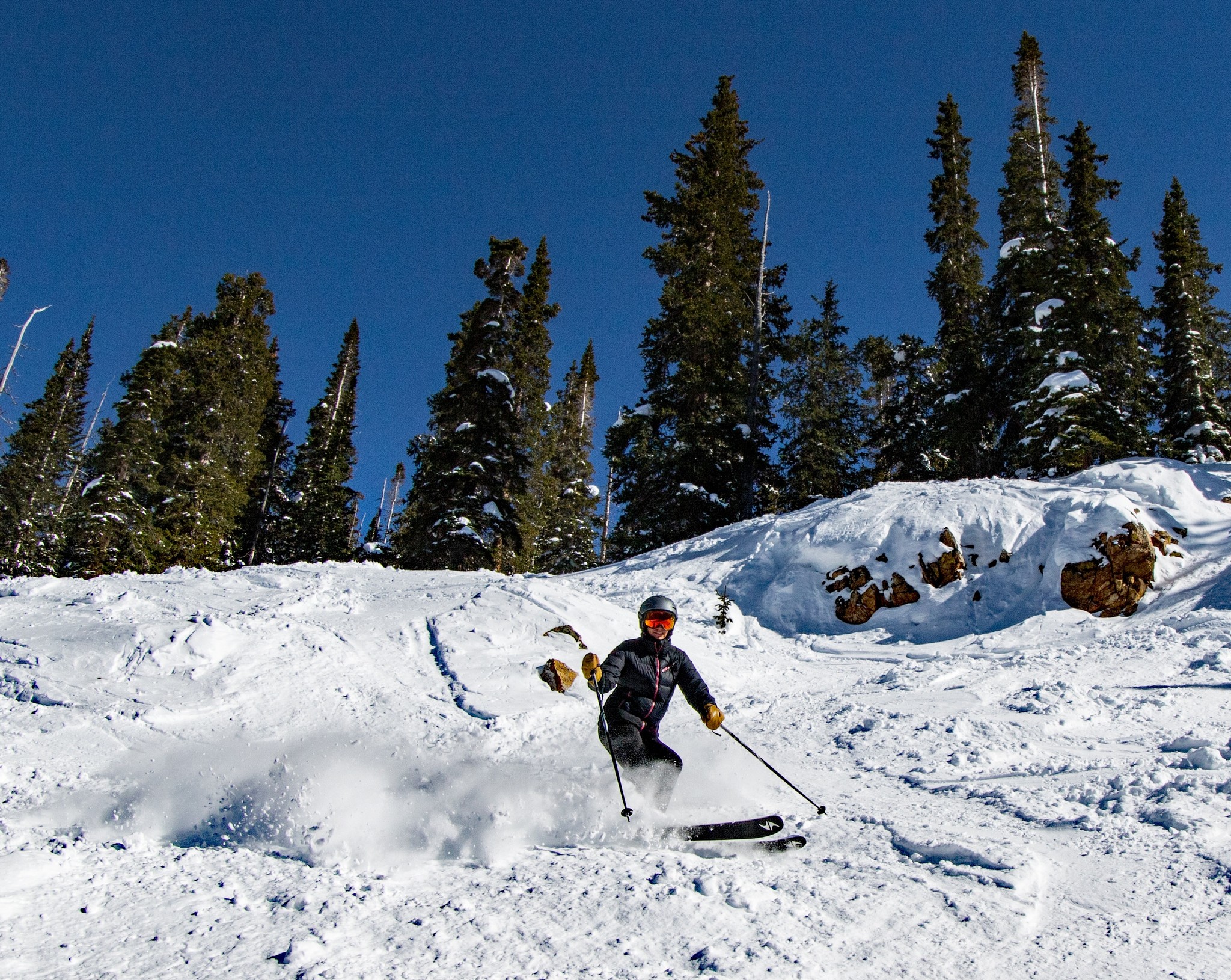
[639,595,677,636]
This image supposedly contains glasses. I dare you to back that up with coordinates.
[642,610,675,631]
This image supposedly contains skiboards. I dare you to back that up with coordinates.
[653,816,806,853]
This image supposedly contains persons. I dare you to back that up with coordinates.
[581,596,726,822]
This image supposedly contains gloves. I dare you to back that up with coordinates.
[700,704,726,730]
[582,653,602,685]
